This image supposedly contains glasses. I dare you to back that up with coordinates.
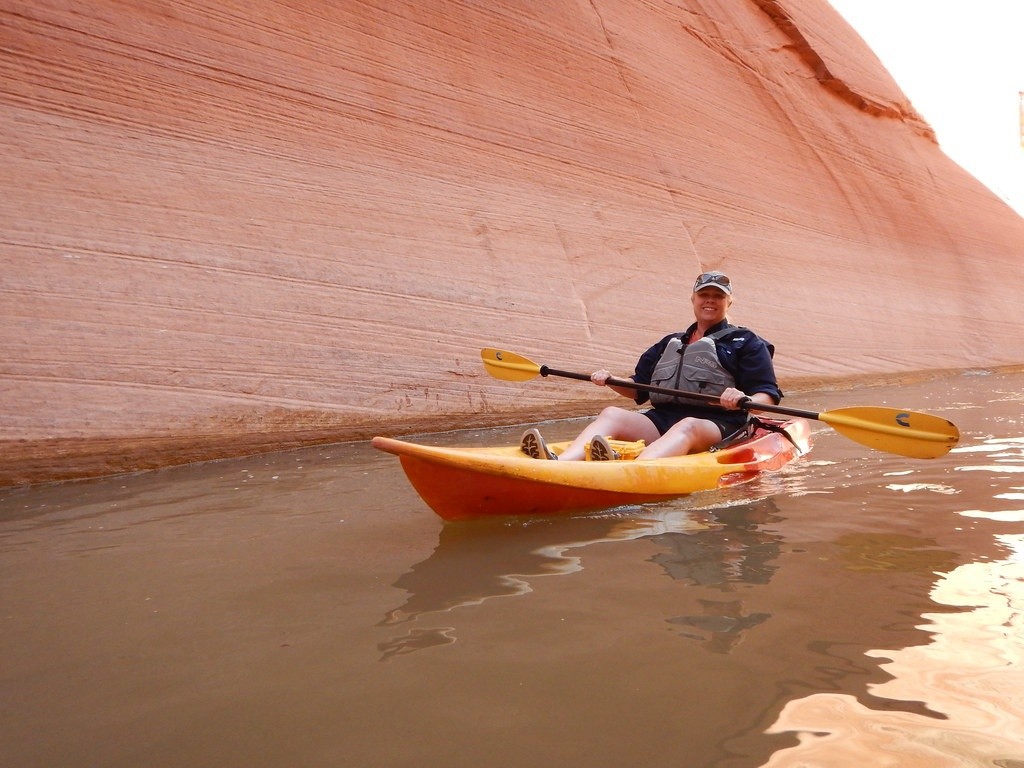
[695,274,729,286]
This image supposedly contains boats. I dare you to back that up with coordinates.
[370,412,814,519]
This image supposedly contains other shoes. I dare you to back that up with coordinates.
[590,435,619,461]
[520,428,558,460]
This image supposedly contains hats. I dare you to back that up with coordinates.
[694,270,733,296]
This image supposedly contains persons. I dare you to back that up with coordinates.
[519,270,784,462]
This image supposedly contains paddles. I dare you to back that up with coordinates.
[480,347,960,460]
